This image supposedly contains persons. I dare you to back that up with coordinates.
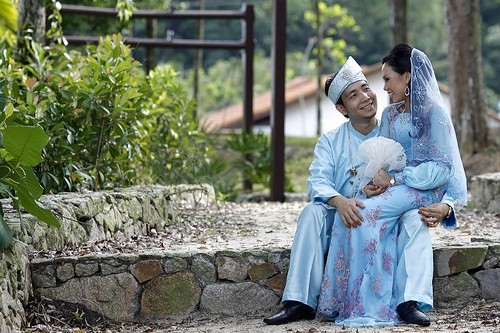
[263,55,458,327]
[315,42,467,327]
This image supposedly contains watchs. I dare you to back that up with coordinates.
[446,204,452,219]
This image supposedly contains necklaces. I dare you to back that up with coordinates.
[400,109,411,125]
[348,126,365,176]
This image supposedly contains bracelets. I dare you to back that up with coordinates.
[390,176,395,186]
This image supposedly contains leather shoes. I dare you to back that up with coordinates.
[395,300,431,328]
[263,300,317,327]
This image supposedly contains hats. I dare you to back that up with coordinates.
[324,53,368,104]
